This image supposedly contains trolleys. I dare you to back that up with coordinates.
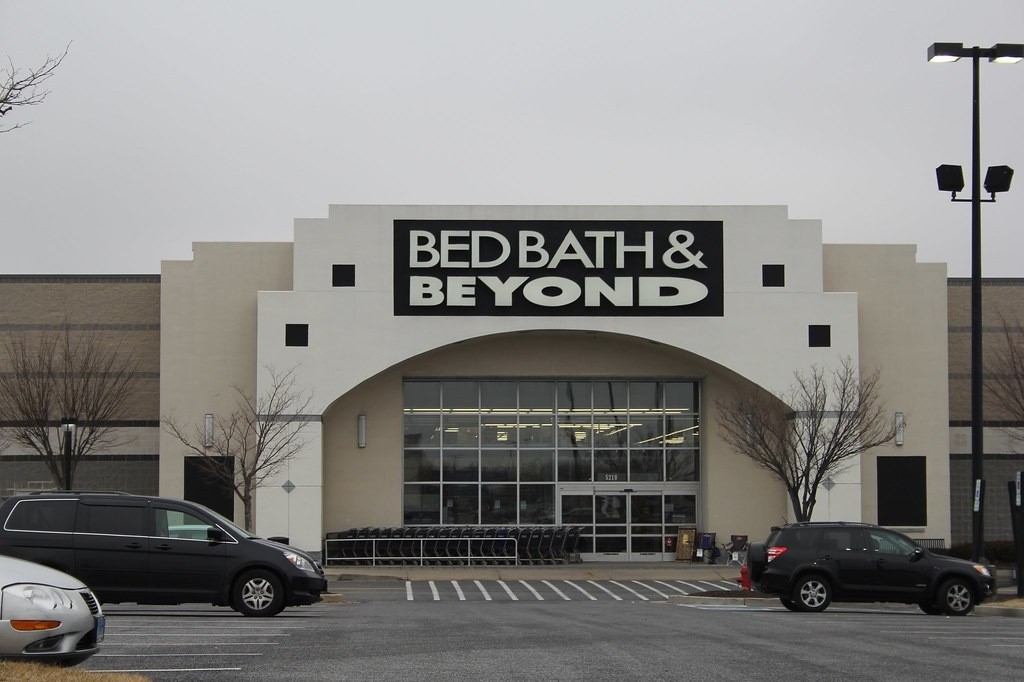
[327,523,584,566]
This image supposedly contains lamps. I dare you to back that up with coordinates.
[204,413,214,447]
[358,415,366,448]
[895,412,903,445]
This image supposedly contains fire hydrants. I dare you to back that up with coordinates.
[736,565,754,594]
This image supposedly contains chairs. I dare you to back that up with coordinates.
[685,532,717,565]
[721,534,752,566]
[871,539,881,552]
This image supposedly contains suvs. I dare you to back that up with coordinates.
[748,521,999,617]
[0,488,328,618]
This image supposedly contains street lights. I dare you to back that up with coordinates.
[926,41,1024,564]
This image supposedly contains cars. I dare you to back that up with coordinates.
[0,554,108,667]
